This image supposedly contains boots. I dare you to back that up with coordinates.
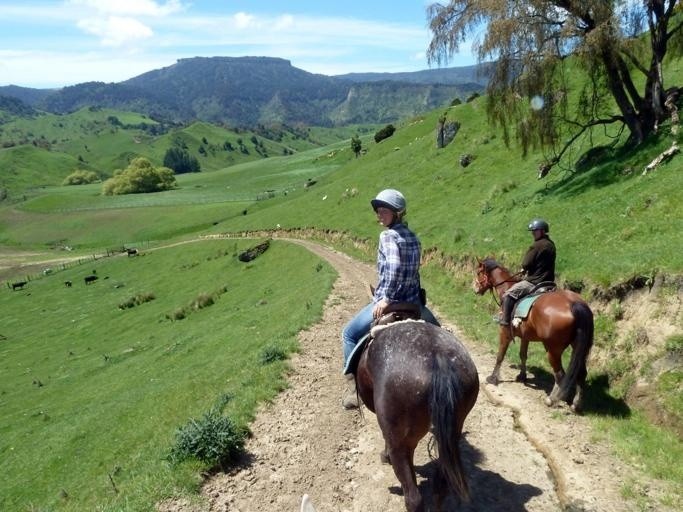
[341,374,363,408]
[499,296,516,326]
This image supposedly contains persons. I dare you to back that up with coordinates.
[340,188,443,408]
[492,218,555,326]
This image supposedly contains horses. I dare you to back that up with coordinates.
[470,255,594,413]
[353,281,480,512]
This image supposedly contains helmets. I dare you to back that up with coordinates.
[527,219,549,233]
[371,188,407,219]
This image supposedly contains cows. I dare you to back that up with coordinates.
[84,275,98,284]
[127,249,139,257]
[12,281,27,290]
[65,281,72,286]
